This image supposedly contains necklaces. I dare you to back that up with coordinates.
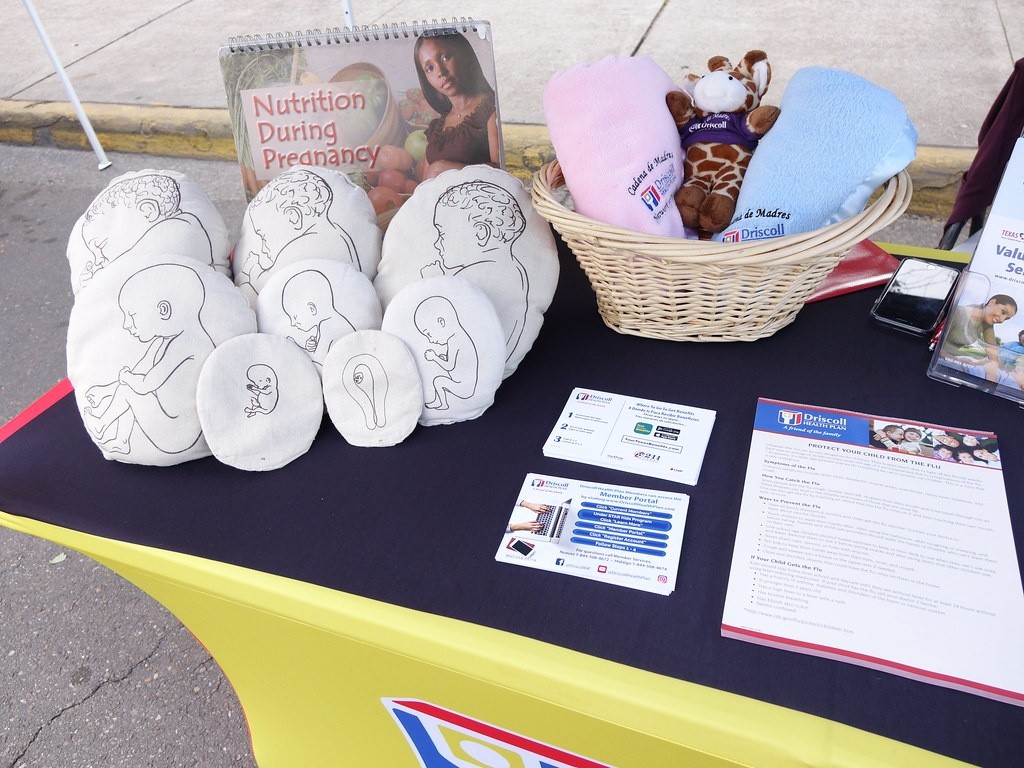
[454,111,466,118]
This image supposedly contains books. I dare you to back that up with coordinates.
[804,239,901,302]
[928,136,1024,403]
[720,397,1024,708]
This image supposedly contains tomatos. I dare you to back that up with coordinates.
[366,128,428,213]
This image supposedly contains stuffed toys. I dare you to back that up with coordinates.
[666,49,781,233]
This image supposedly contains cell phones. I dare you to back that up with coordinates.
[509,539,535,559]
[871,257,961,337]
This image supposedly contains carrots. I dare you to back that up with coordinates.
[239,162,269,195]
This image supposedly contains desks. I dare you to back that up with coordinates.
[0,243,1024,768]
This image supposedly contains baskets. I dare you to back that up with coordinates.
[328,62,405,148]
[405,114,441,131]
[531,157,914,342]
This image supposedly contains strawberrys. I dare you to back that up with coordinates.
[398,88,438,125]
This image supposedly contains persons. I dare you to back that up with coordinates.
[869,421,1001,470]
[414,29,498,168]
[938,294,1024,392]
[504,500,552,533]
